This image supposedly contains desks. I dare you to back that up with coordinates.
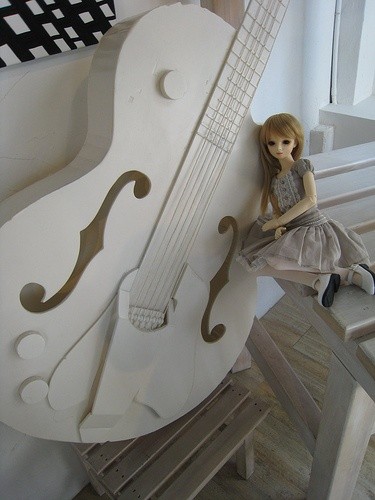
[228,139,375,500]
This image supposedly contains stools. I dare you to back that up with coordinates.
[71,376,271,499]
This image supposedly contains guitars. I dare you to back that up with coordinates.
[2,1,286,443]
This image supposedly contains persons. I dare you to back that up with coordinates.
[234,113,375,308]
